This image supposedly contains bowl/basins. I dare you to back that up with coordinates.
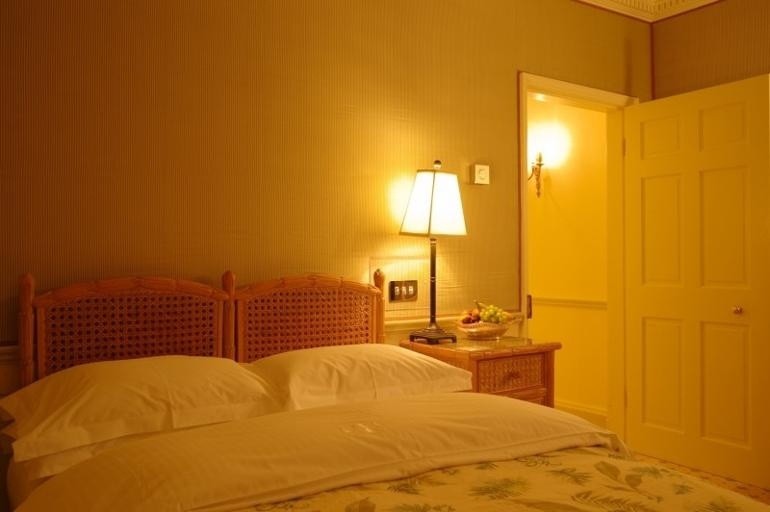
[449,313,524,341]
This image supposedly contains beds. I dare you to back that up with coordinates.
[14,267,770,508]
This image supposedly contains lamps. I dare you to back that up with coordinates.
[399,160,468,345]
[527,126,573,197]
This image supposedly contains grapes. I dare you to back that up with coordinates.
[472,300,512,323]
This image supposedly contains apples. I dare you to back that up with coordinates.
[462,310,480,324]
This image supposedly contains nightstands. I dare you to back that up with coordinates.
[400,339,562,409]
